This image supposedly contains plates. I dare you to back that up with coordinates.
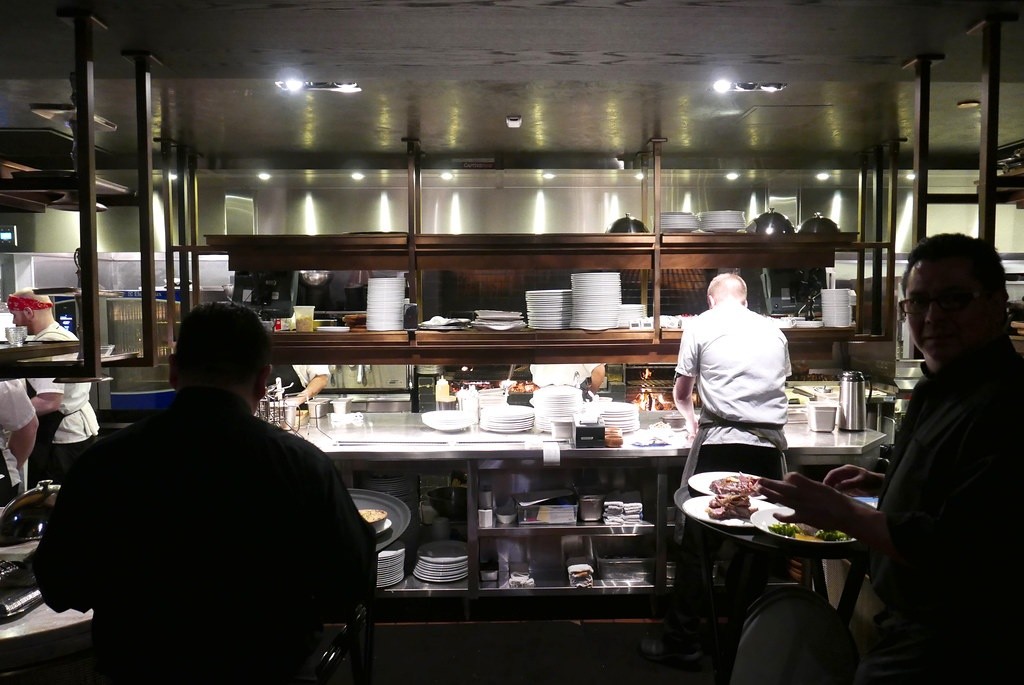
[781,289,853,329]
[533,385,642,433]
[411,540,469,583]
[681,471,879,543]
[421,404,534,433]
[470,309,528,332]
[362,471,420,529]
[366,277,406,331]
[527,272,647,331]
[368,517,392,536]
[316,326,351,333]
[376,540,406,588]
[650,210,748,233]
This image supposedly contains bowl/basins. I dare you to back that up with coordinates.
[4,326,28,345]
[495,507,518,523]
[313,319,337,331]
[604,427,623,447]
[101,345,115,357]
[426,486,467,519]
[579,494,605,521]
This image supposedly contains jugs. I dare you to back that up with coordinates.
[836,371,873,432]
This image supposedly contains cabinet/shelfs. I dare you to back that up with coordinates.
[205,228,861,362]
[466,450,667,601]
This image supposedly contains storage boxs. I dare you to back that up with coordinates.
[103,297,181,393]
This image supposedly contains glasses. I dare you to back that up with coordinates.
[899,290,985,314]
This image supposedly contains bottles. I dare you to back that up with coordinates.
[434,375,478,411]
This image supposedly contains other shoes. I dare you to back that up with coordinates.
[640,638,703,661]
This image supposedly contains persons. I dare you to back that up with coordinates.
[0,378,40,515]
[267,364,331,411]
[34,299,377,684]
[757,232,1024,685]
[530,363,606,402]
[640,274,792,659]
[6,289,101,485]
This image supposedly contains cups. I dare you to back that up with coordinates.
[431,517,450,540]
[651,314,696,328]
[405,303,418,329]
[293,305,315,332]
[778,318,796,328]
